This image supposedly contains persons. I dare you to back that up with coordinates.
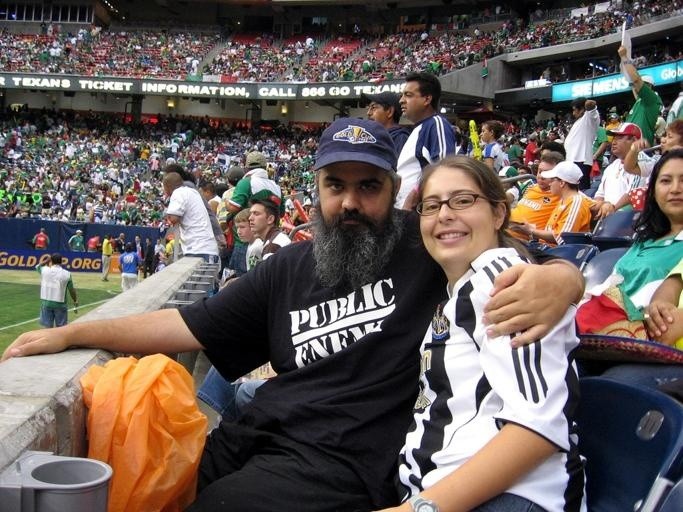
[0,118,586,510]
[369,156,591,511]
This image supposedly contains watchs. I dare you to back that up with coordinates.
[407,494,439,510]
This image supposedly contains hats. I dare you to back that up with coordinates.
[497,166,519,178]
[245,152,268,169]
[225,166,244,181]
[640,75,655,86]
[540,161,584,185]
[361,91,403,118]
[605,123,642,140]
[313,117,399,174]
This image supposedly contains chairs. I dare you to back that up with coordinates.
[0,2,682,83]
[516,199,683,512]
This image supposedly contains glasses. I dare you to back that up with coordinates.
[416,193,491,217]
